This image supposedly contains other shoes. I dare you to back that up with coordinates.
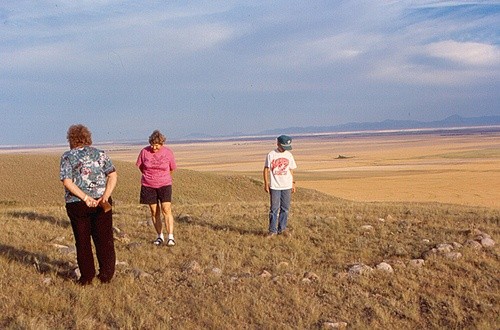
[265,231,277,238]
[279,230,291,236]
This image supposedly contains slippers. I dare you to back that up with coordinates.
[152,238,164,245]
[168,239,176,246]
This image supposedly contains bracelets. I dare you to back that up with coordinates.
[291,181,296,184]
[82,193,89,202]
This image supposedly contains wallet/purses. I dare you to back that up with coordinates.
[99,200,112,213]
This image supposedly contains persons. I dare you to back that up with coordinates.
[263,134,297,239]
[59,123,119,287]
[136,129,177,247]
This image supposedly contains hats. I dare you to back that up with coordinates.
[276,135,293,150]
